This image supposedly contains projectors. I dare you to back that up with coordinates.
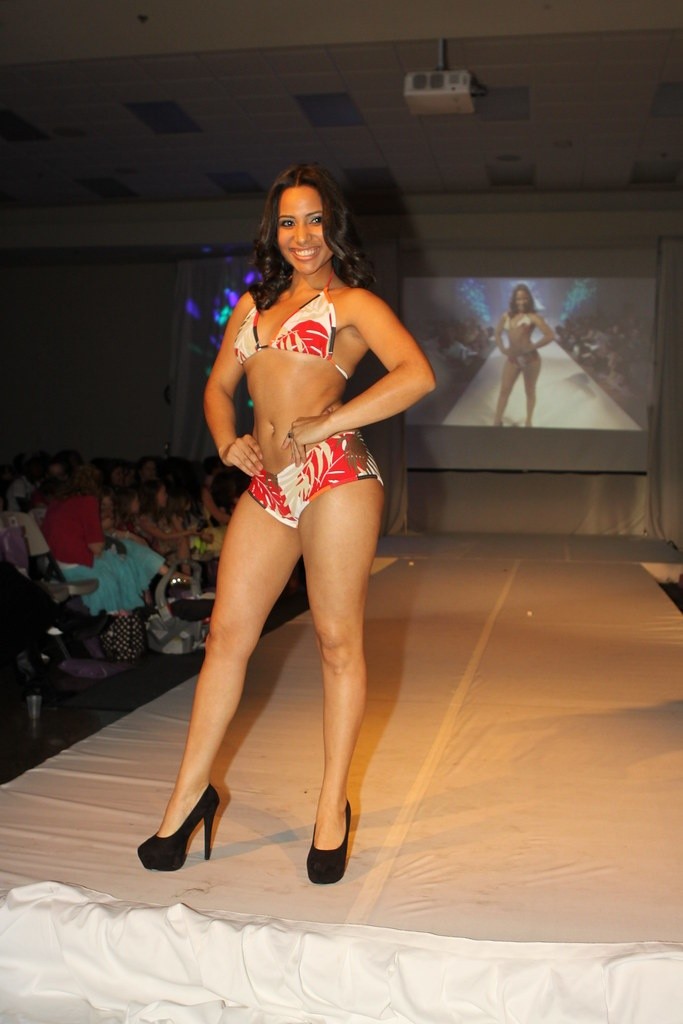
[403,69,476,115]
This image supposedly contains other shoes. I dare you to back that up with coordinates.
[22,676,77,704]
[169,574,195,585]
[57,608,83,639]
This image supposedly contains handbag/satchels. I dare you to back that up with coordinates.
[101,616,144,662]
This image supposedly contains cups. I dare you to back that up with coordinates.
[27,695,42,719]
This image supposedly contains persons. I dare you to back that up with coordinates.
[0,450,298,695]
[139,165,439,883]
[418,312,496,390]
[491,284,553,426]
[555,313,656,398]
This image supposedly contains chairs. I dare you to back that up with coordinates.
[2,510,105,660]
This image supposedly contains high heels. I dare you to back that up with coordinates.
[306,799,351,884]
[137,783,219,871]
